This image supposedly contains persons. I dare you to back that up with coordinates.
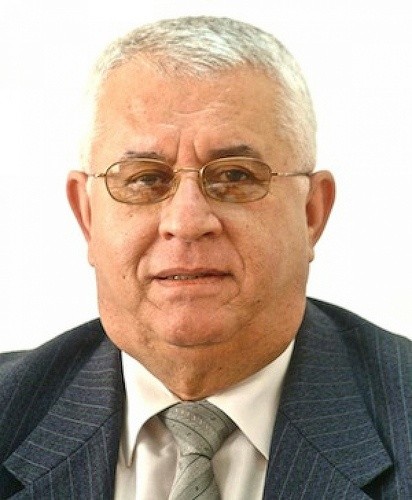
[0,15,412,500]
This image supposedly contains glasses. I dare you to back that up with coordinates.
[86,159,309,202]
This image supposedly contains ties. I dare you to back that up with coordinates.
[157,400,238,500]
